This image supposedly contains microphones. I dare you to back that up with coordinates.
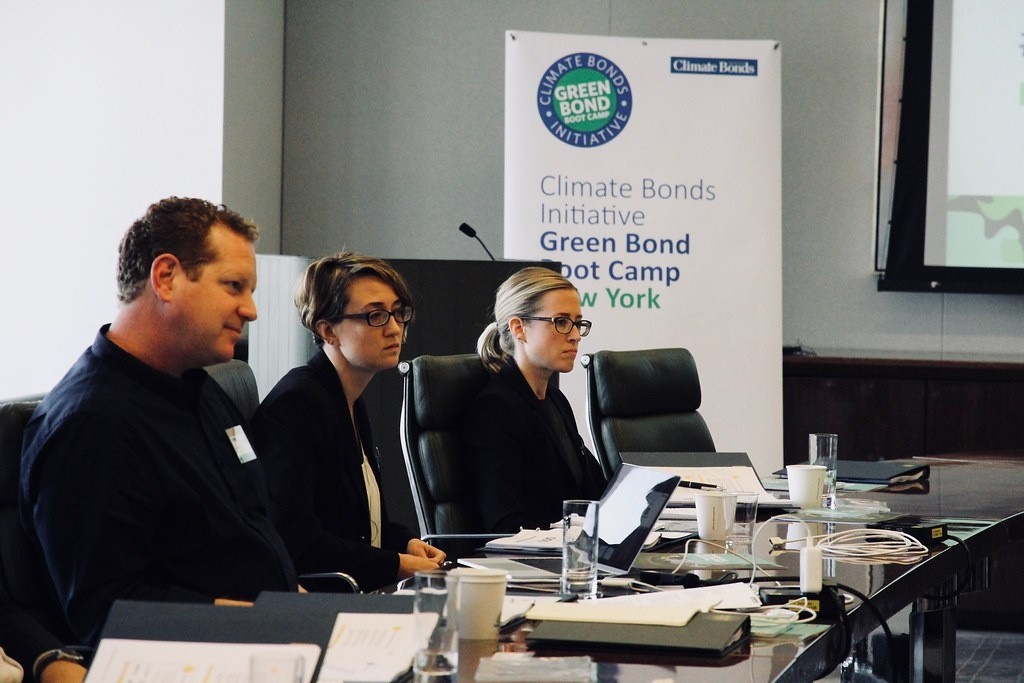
[458,222,495,259]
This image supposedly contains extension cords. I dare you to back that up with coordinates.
[763,589,844,617]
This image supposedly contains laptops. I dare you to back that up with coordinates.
[456,463,680,580]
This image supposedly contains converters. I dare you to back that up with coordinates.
[866,517,948,545]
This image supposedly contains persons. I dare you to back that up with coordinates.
[455,267,610,547]
[21,197,306,643]
[241,252,446,592]
[0,580,96,683]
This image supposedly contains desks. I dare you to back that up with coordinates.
[213,458,1024,683]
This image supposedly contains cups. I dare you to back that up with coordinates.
[785,523,824,551]
[694,492,737,541]
[786,464,826,509]
[721,490,759,545]
[695,540,725,583]
[561,500,600,596]
[822,523,837,578]
[809,432,838,509]
[445,567,508,639]
[410,570,460,675]
[725,543,755,579]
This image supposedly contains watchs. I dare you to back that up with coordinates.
[35,648,84,683]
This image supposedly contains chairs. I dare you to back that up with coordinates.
[0,394,39,606]
[397,355,519,553]
[580,346,716,476]
[207,359,260,412]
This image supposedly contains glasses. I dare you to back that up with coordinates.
[332,305,414,327]
[507,317,592,337]
